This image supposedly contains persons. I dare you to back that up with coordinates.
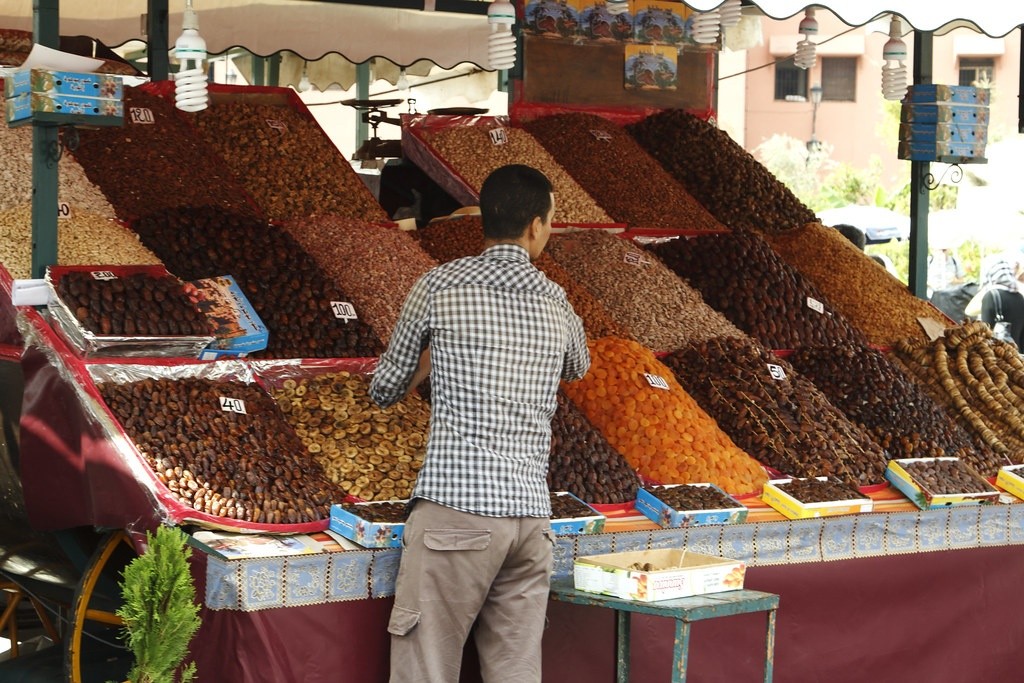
[832,224,1024,358]
[378,107,488,228]
[369,164,591,683]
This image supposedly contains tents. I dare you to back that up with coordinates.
[0,0,498,93]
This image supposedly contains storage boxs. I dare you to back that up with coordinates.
[60,34,149,87]
[897,84,991,161]
[330,498,409,549]
[184,274,268,360]
[550,491,606,537]
[996,464,1024,501]
[883,456,1001,511]
[5,69,123,123]
[574,548,746,602]
[634,481,747,528]
[762,475,873,520]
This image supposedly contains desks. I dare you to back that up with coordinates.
[550,583,780,683]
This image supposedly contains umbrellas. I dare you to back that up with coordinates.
[815,207,911,245]
